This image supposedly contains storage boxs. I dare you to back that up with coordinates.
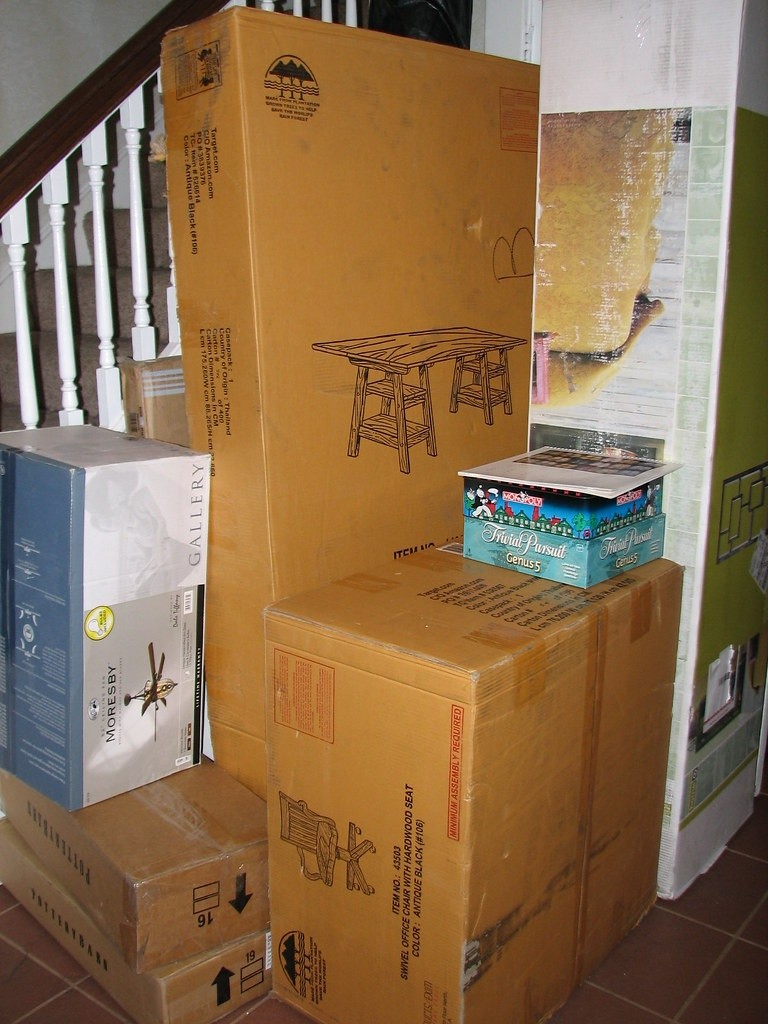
[0,5,683,1024]
